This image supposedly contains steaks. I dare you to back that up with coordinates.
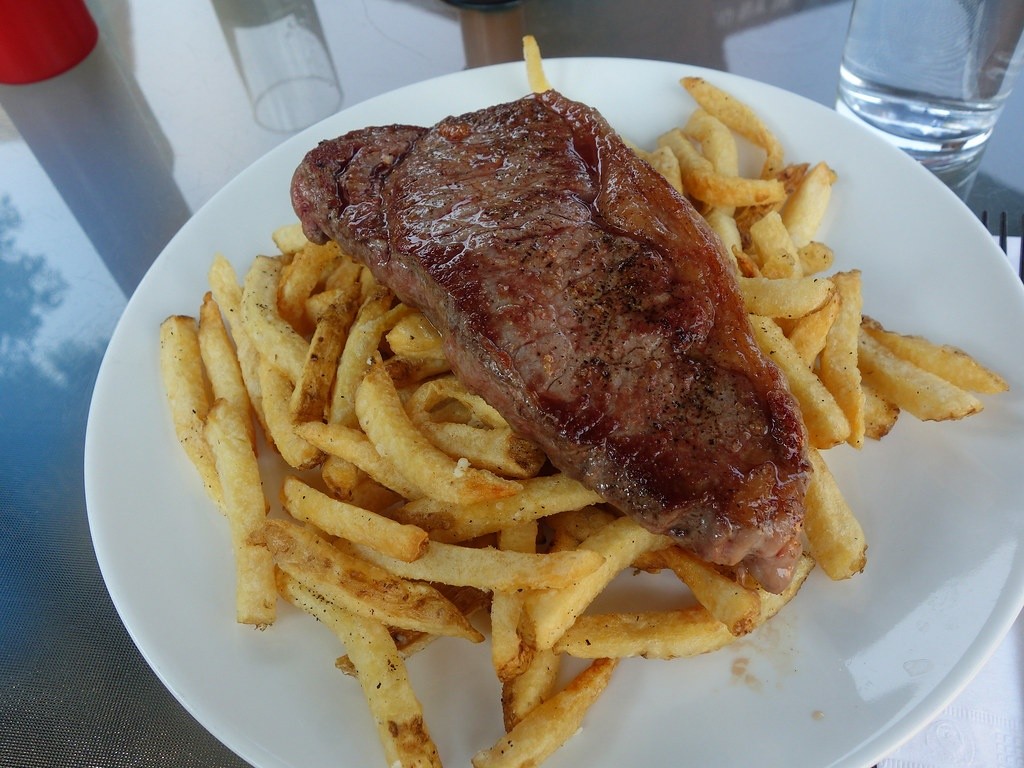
[287,91,803,566]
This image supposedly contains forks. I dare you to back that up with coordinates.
[980,207,1023,285]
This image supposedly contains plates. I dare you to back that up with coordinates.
[82,56,1023,768]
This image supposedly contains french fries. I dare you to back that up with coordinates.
[159,33,1014,768]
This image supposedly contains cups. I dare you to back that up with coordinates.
[834,1,1024,185]
